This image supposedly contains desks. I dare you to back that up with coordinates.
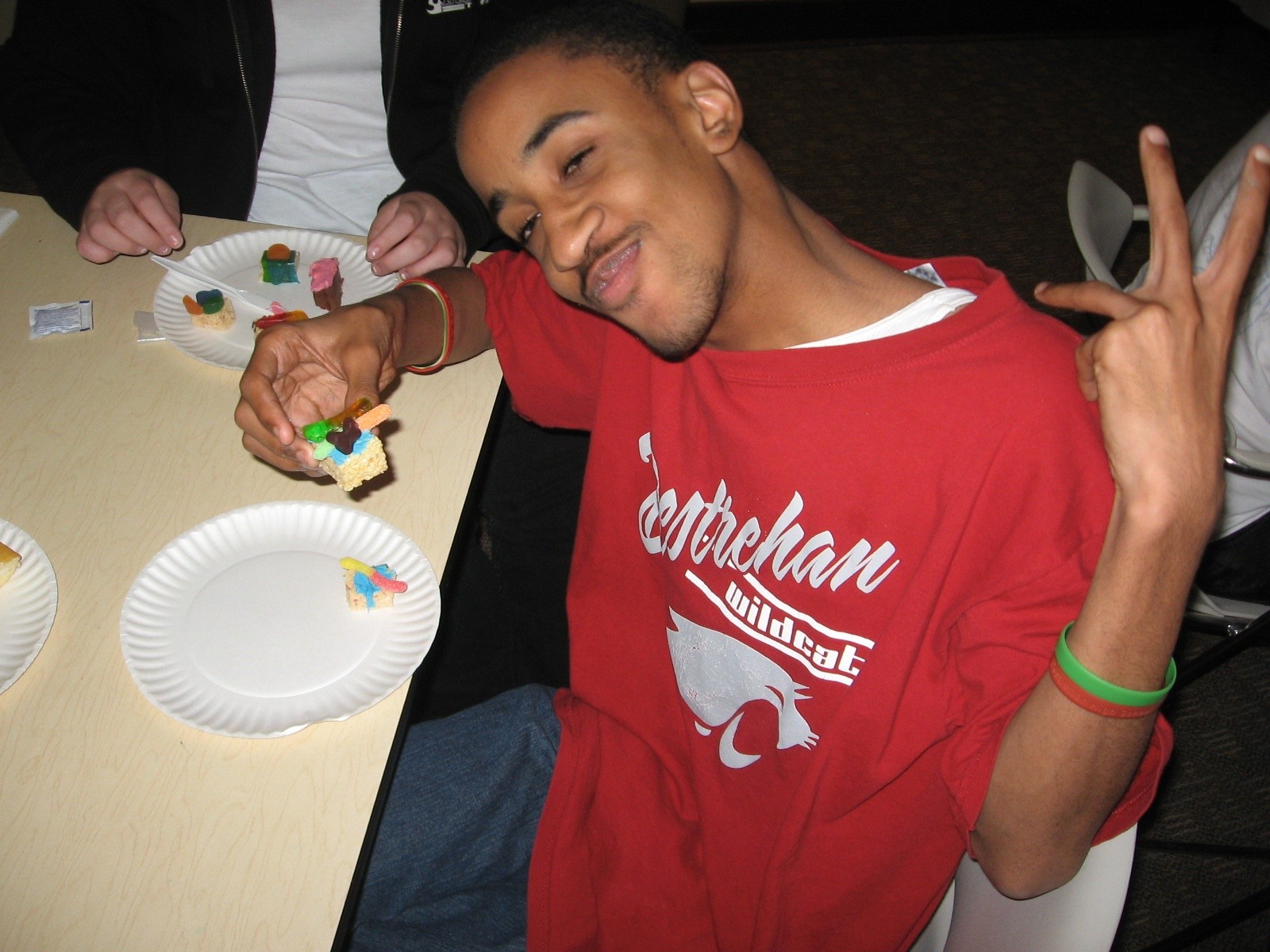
[0,187,503,952]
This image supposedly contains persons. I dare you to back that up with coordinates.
[1120,114,1269,606]
[1,1,520,282]
[233,26,1270,951]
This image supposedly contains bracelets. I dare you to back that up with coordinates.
[1048,621,1177,719]
[394,277,454,375]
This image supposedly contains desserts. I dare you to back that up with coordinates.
[309,256,343,313]
[339,556,408,612]
[183,288,236,330]
[252,309,310,344]
[261,243,297,285]
[302,398,392,492]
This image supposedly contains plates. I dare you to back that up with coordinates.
[120,500,441,739]
[152,228,400,372]
[0,517,58,696]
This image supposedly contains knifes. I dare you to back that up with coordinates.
[151,254,286,314]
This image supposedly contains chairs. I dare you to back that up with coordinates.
[906,821,1136,952]
